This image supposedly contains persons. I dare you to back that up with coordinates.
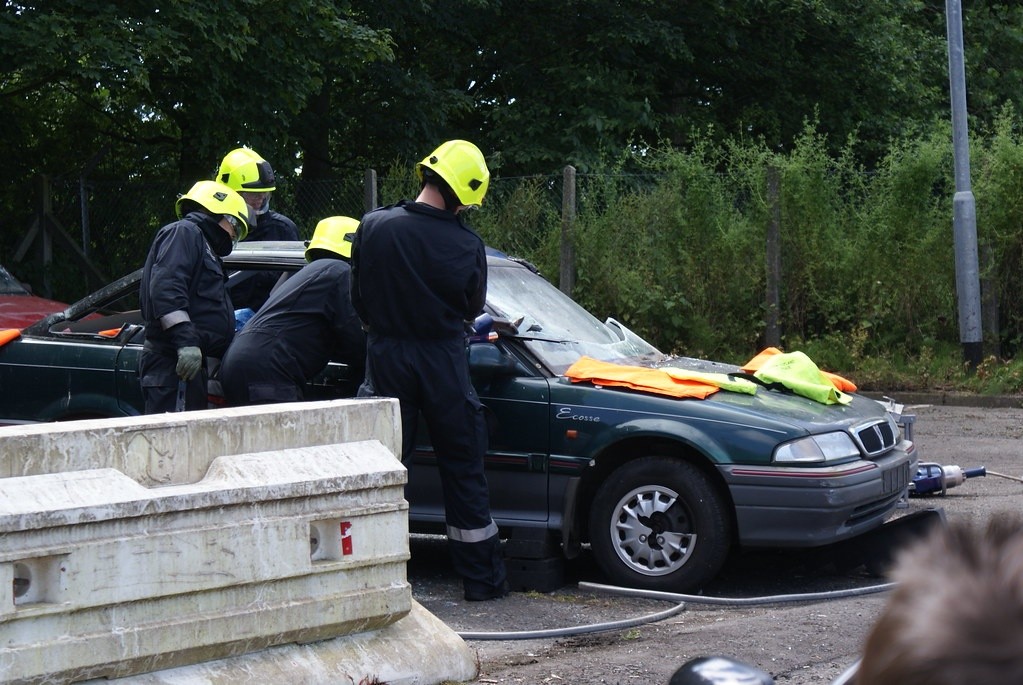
[220,214,366,407]
[216,148,302,310]
[860,506,1023,685]
[349,140,511,603]
[138,179,248,415]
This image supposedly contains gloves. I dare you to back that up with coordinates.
[176,345,202,381]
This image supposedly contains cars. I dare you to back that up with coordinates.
[0,239,921,596]
[1,256,113,339]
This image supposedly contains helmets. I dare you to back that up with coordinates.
[416,140,490,207]
[176,180,248,240]
[216,148,276,192]
[303,216,360,263]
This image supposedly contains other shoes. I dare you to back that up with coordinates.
[464,580,509,602]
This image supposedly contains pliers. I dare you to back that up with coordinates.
[471,316,528,356]
